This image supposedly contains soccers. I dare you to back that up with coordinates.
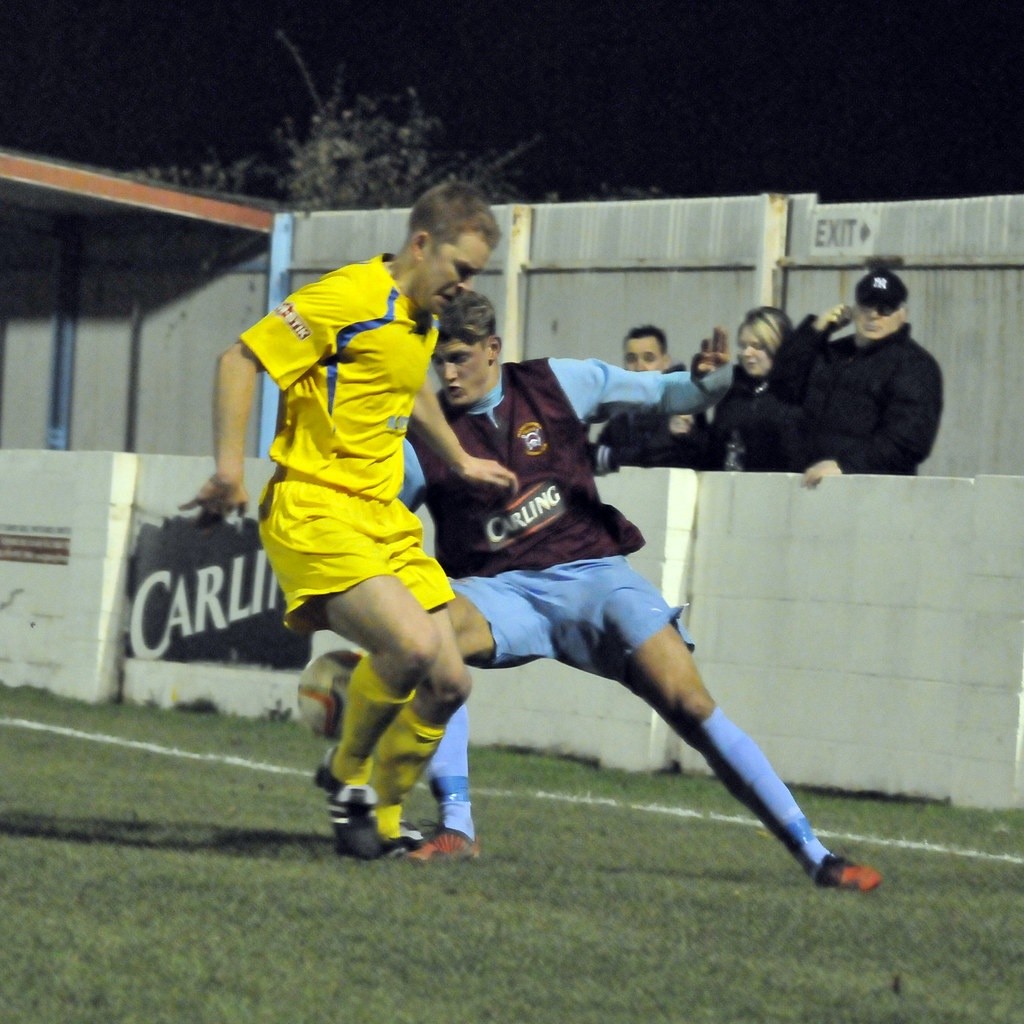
[297,648,364,741]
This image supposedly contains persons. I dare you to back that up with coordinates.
[398,288,880,893]
[597,267,943,490]
[178,181,520,862]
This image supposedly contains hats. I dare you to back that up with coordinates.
[854,271,907,307]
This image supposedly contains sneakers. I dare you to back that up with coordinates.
[315,746,384,856]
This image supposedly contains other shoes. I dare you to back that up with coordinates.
[403,826,479,860]
[815,853,883,890]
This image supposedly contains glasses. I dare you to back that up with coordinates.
[861,302,900,315]
